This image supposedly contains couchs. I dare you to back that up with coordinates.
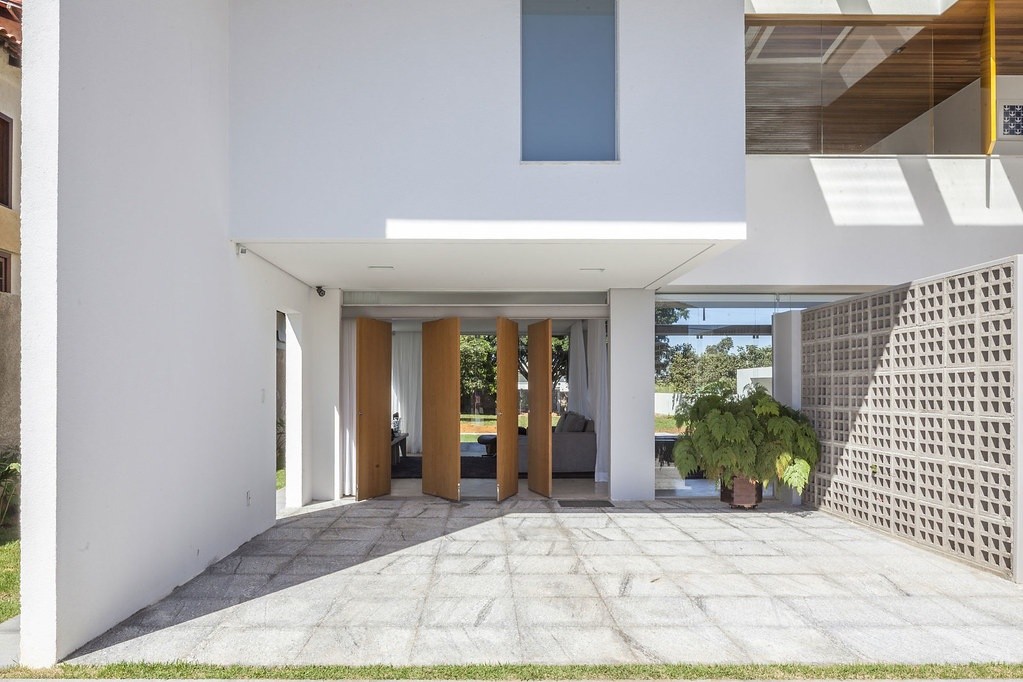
[518,420,596,474]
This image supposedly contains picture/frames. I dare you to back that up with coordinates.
[997,99,1023,141]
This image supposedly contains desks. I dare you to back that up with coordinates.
[390,433,409,463]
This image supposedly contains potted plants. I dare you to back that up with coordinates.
[670,382,822,508]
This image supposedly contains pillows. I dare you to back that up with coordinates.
[555,412,566,432]
[564,413,587,432]
